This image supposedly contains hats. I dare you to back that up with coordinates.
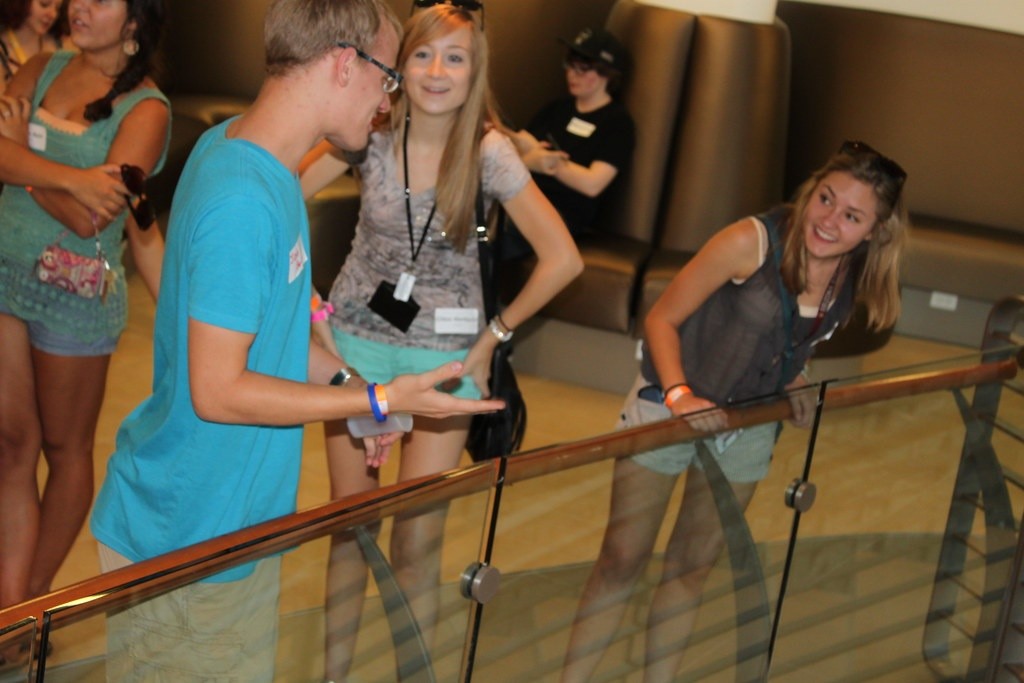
[558,27,627,69]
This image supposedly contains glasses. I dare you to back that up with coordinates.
[563,59,607,74]
[120,164,156,232]
[410,0,484,31]
[837,141,908,209]
[338,41,405,93]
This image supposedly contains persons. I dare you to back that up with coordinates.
[293,7,588,683]
[484,24,635,325]
[552,134,907,683]
[1,0,82,101]
[85,2,511,683]
[0,0,175,674]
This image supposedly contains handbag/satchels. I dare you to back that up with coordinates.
[465,342,527,463]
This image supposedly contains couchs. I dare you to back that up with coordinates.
[640,18,794,345]
[765,1,1022,353]
[511,2,693,335]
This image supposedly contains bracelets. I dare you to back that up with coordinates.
[330,367,359,389]
[373,382,390,418]
[308,304,333,322]
[308,293,322,312]
[367,381,387,424]
